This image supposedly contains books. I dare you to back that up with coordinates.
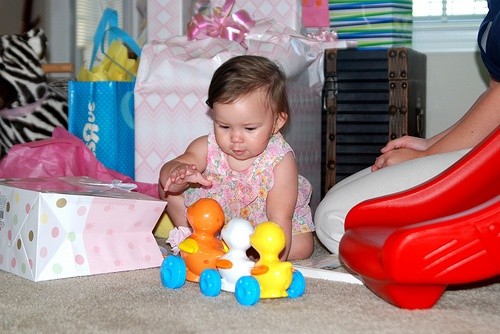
[291,255,366,285]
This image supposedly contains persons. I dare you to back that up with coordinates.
[315,0,500,255]
[158,55,316,262]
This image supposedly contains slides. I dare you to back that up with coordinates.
[338,124,500,310]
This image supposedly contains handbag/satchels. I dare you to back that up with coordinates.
[0,175,167,283]
[67,7,141,182]
[0,126,160,199]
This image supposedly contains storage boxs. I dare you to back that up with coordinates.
[321,47,427,200]
[134,17,320,225]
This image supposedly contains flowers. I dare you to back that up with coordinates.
[301,25,338,43]
[187,0,256,50]
